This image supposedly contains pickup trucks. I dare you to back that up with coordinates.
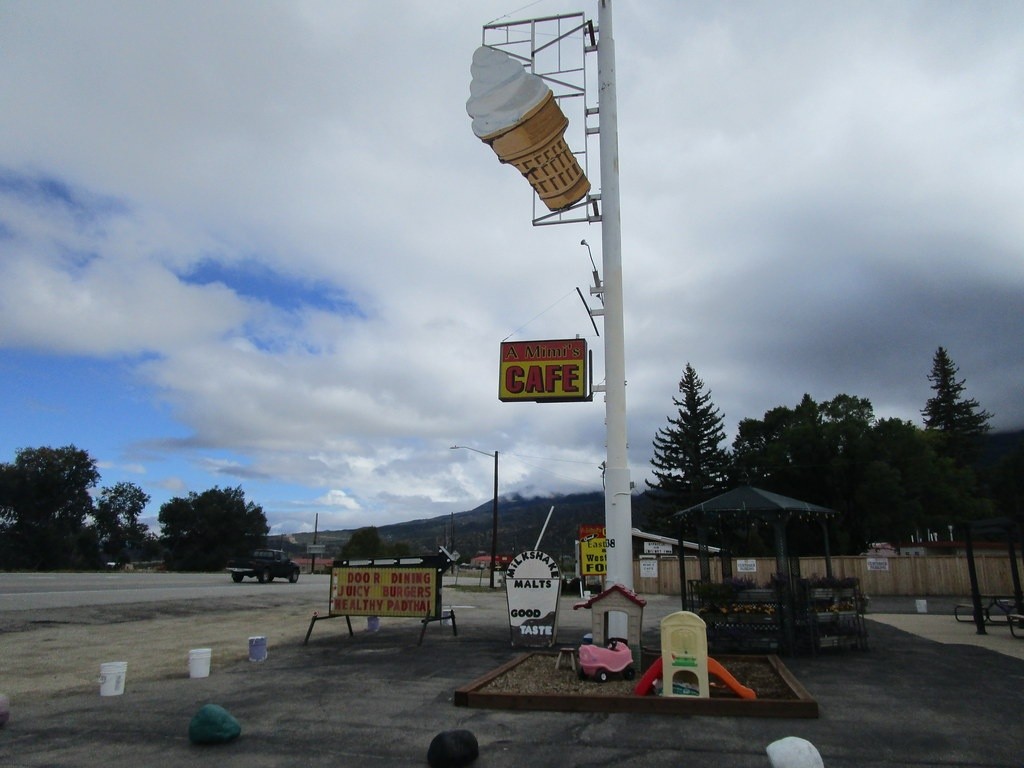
[224,549,300,584]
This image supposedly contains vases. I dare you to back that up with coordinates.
[820,634,858,647]
[706,611,774,625]
[733,587,776,604]
[815,610,857,623]
[810,588,854,600]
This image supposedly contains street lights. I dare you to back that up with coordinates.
[449,445,499,588]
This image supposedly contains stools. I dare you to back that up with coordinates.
[554,646,576,671]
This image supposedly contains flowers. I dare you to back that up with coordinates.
[698,572,860,641]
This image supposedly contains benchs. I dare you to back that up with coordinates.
[953,602,1024,639]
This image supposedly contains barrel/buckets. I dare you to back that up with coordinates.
[584,591,591,600]
[368,618,380,632]
[248,636,267,661]
[189,648,211,677]
[916,600,927,613]
[443,611,454,626]
[101,662,127,696]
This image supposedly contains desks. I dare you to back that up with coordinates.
[981,594,1015,624]
